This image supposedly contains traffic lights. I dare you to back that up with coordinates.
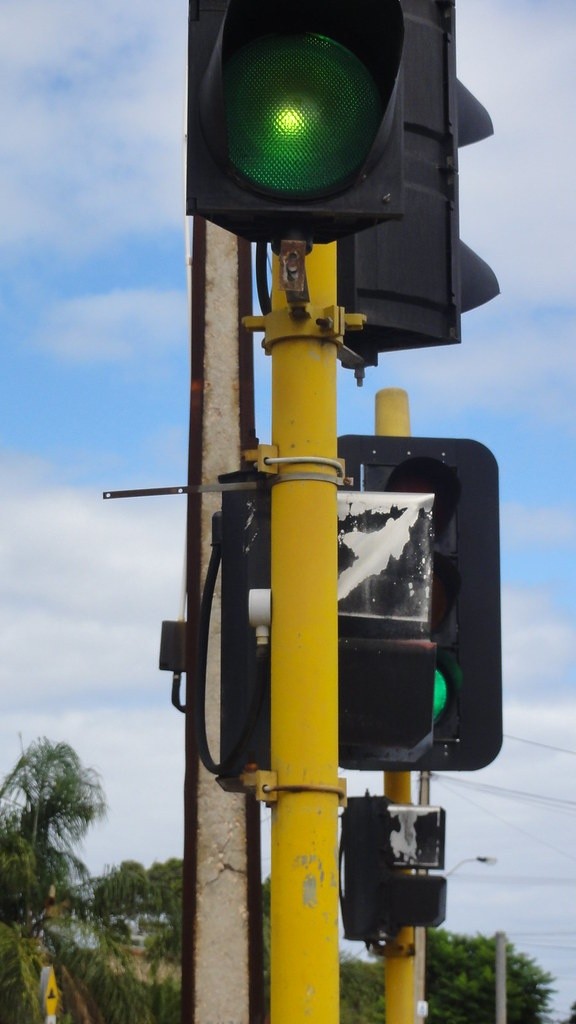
[189,0,407,247]
[328,433,505,772]
